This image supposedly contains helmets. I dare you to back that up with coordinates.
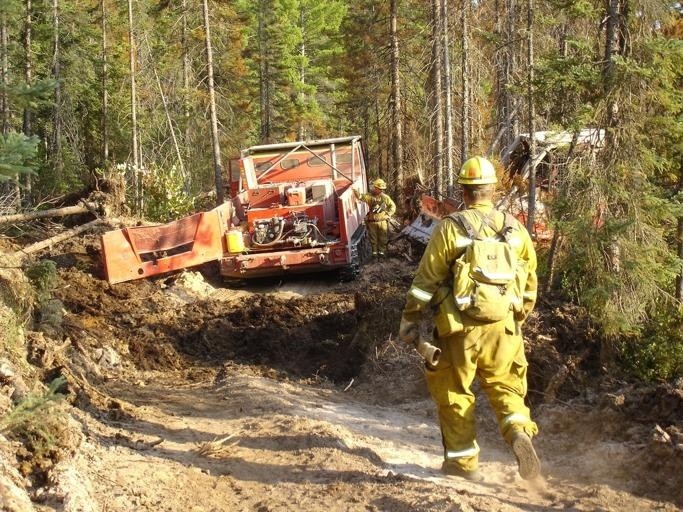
[458,157,499,185]
[373,179,386,190]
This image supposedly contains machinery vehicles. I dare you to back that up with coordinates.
[402,128,608,262]
[100,135,369,288]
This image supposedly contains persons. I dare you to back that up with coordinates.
[399,155,540,481]
[352,178,396,263]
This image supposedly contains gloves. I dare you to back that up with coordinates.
[399,317,441,367]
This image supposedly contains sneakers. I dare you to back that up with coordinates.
[510,432,540,480]
[442,461,481,481]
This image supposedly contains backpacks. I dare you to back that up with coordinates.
[442,211,517,322]
